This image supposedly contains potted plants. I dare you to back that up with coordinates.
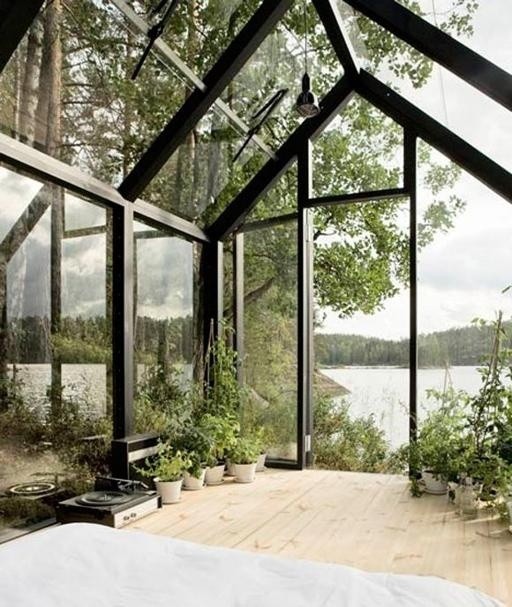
[131,319,275,503]
[391,387,512,533]
